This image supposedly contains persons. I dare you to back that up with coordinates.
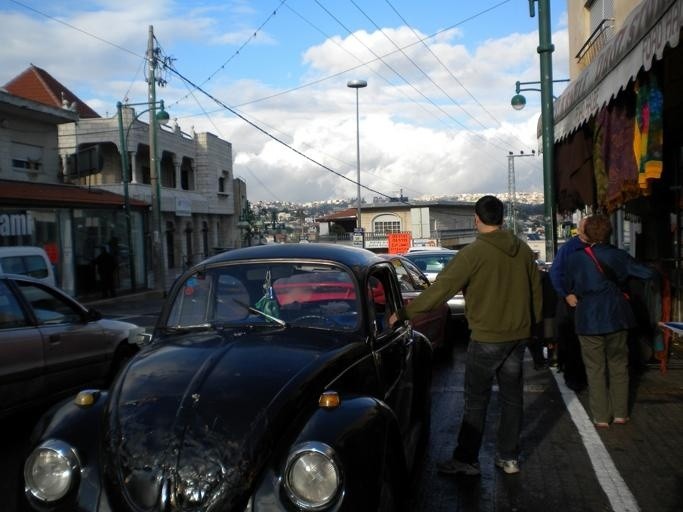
[547,218,588,391]
[388,195,542,477]
[572,215,657,427]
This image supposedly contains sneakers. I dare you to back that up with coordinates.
[613,415,631,424]
[494,451,520,474]
[592,416,610,428]
[435,458,482,476]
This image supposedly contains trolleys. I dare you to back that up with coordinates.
[175,256,200,297]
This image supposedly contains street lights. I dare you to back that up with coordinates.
[510,75,570,110]
[346,79,366,247]
[115,100,170,293]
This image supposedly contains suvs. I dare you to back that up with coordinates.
[521,221,544,235]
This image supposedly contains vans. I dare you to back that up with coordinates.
[0,246,55,310]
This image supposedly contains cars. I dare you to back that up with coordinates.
[271,253,451,351]
[0,274,144,422]
[407,247,449,254]
[399,249,466,316]
[209,274,244,293]
[23,243,436,511]
